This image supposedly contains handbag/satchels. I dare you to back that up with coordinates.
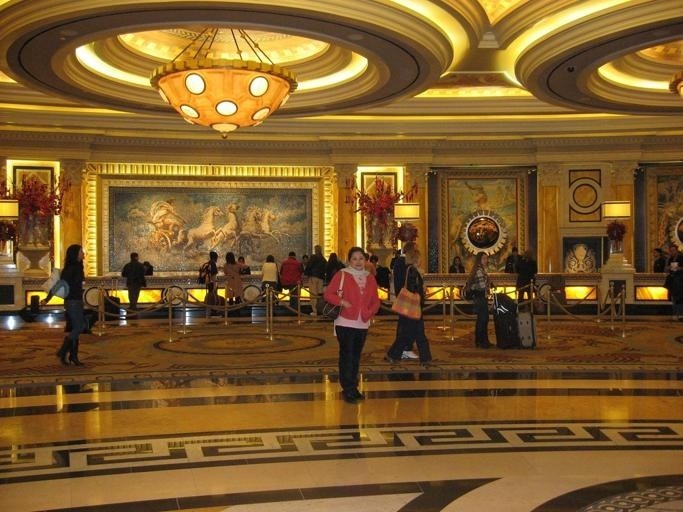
[317,270,345,319]
[205,294,224,305]
[460,266,480,300]
[276,263,281,290]
[41,269,64,298]
[391,265,422,321]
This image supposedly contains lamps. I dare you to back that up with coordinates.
[148,27,297,140]
[0,199,19,220]
[601,199,630,220]
[392,202,420,221]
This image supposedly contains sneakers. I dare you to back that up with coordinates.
[401,351,419,359]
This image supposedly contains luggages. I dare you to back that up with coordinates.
[517,310,538,350]
[491,287,518,349]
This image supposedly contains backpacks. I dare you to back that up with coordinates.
[198,262,211,284]
[493,292,517,316]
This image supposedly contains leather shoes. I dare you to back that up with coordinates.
[353,388,365,400]
[344,393,356,403]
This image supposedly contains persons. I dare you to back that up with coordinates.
[260,242,439,404]
[449,256,466,273]
[122,253,147,311]
[150,196,189,234]
[504,246,522,273]
[143,261,153,276]
[204,252,251,316]
[468,251,495,348]
[653,244,683,314]
[40,244,85,369]
[514,250,537,314]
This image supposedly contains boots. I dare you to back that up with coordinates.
[69,340,84,366]
[55,336,69,364]
